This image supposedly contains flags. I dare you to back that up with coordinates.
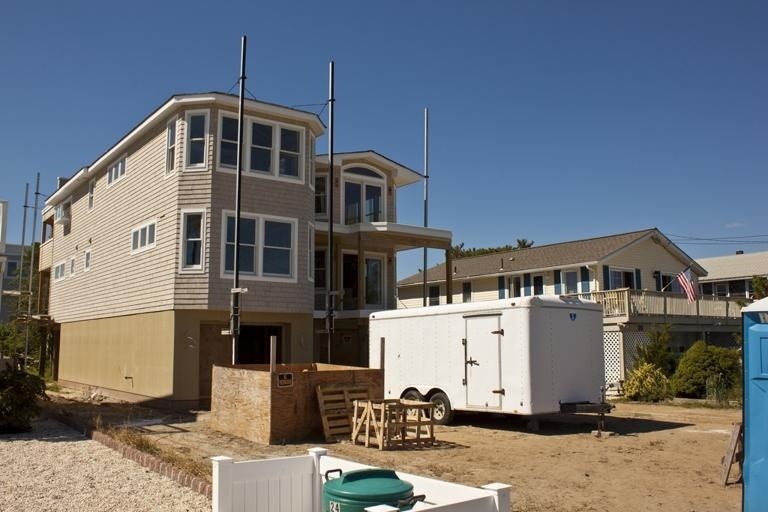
[676,264,699,303]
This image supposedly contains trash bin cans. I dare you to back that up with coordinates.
[321,468,426,512]
